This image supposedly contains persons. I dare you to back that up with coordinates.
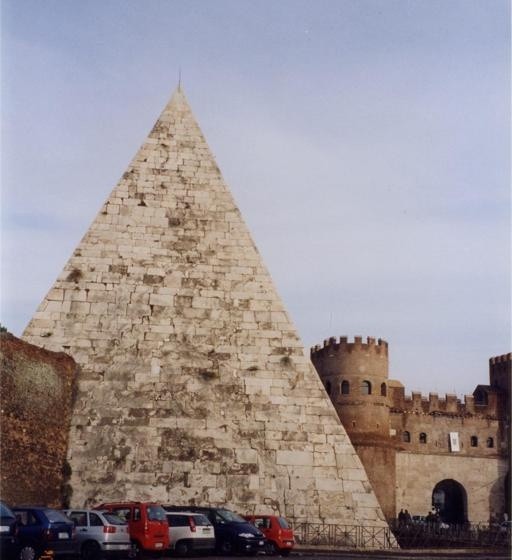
[434,513,442,524]
[398,508,405,528]
[404,509,411,522]
[426,511,434,523]
[431,507,436,515]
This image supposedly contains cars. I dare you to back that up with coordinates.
[0,499,295,559]
[412,514,448,528]
